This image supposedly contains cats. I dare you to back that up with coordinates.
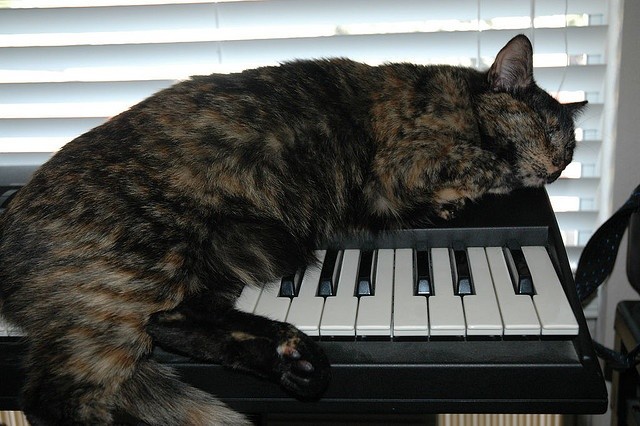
[0,33,590,425]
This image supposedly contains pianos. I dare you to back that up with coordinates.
[0,183,609,426]
